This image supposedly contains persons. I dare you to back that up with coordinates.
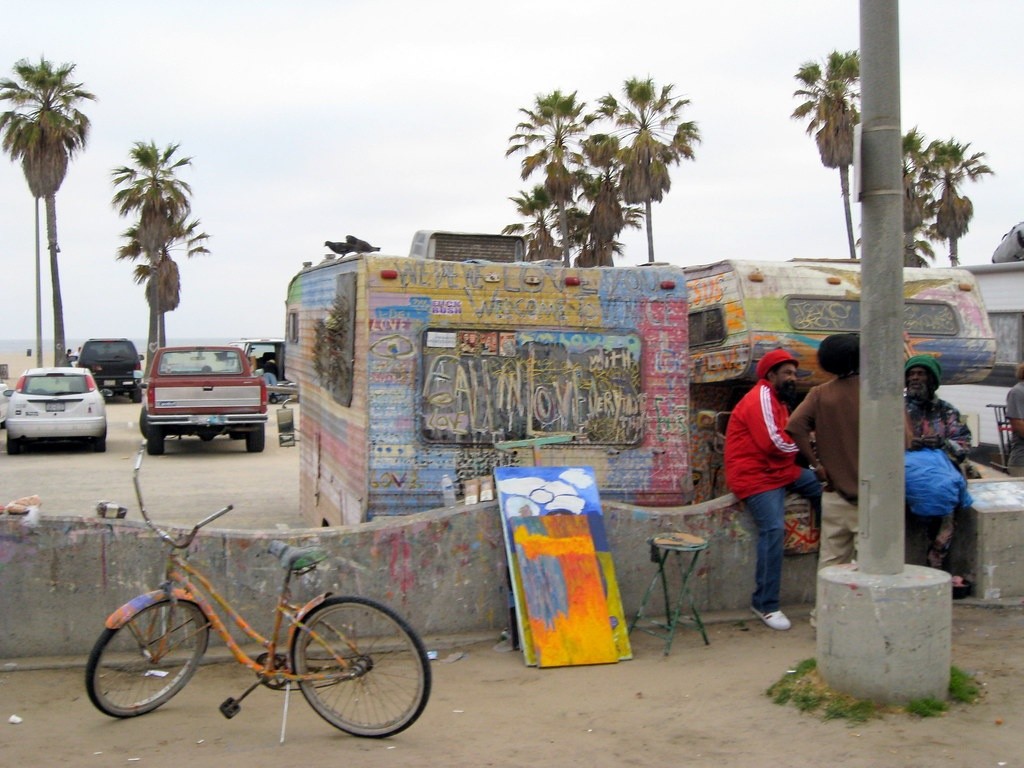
[724,349,823,630]
[782,332,860,626]
[904,355,982,599]
[262,360,277,386]
[66,349,71,357]
[1005,362,1024,478]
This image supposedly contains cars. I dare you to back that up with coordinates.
[3,367,108,454]
[0,364,12,429]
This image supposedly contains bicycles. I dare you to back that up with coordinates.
[83,438,433,744]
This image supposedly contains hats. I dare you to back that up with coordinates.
[905,354,942,391]
[754,349,799,378]
[817,335,859,376]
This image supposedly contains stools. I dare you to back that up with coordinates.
[628,532,710,656]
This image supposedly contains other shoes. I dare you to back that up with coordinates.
[951,575,968,589]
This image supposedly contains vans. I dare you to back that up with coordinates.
[76,338,144,404]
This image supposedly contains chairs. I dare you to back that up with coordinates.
[986,404,1014,474]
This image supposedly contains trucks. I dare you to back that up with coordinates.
[221,338,285,386]
[276,230,693,528]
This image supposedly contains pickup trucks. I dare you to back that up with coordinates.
[139,346,267,456]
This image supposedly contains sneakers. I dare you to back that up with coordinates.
[751,604,791,630]
